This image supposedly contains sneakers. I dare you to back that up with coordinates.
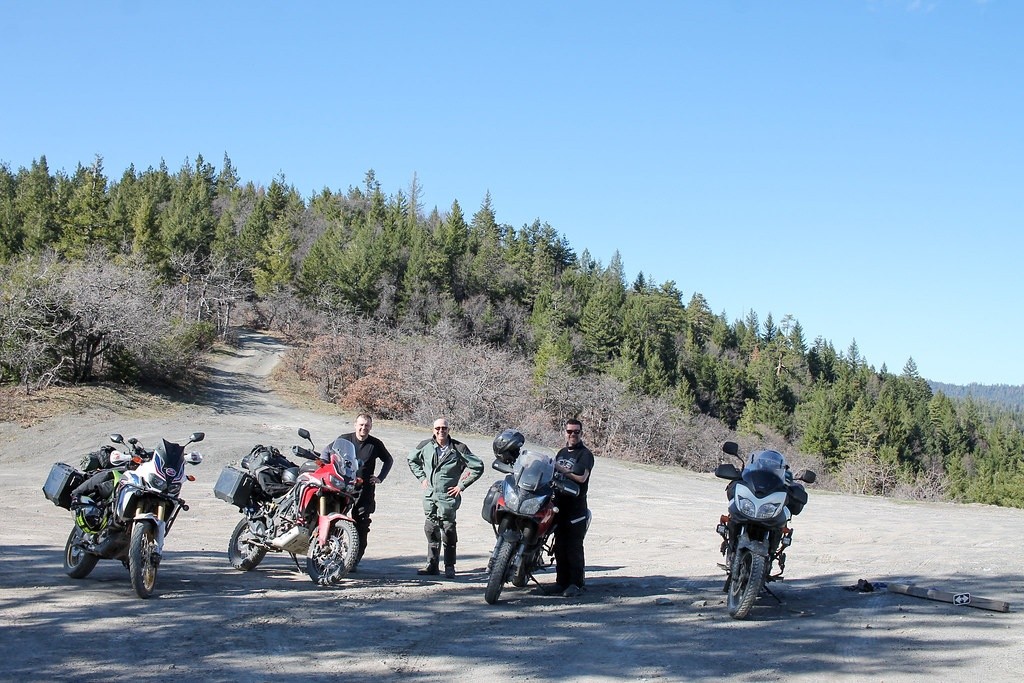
[544,583,582,596]
[417,563,440,575]
[444,564,455,578]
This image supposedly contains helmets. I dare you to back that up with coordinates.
[282,467,299,486]
[69,493,110,534]
[756,450,786,480]
[493,429,525,467]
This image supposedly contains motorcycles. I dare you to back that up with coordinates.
[42,432,205,599]
[483,444,581,605]
[212,428,368,586]
[715,442,817,621]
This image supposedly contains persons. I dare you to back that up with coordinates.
[544,419,594,597]
[406,418,485,579]
[321,414,394,572]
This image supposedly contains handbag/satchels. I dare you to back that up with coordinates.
[241,443,300,496]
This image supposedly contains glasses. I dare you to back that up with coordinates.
[434,426,449,430]
[566,430,581,435]
